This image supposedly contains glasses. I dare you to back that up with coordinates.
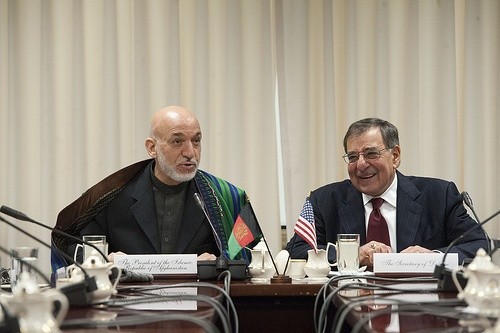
[342,145,389,165]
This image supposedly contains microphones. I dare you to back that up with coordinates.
[0,205,119,281]
[192,192,247,281]
[461,191,491,256]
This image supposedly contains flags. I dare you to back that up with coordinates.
[227,199,261,260]
[293,198,318,256]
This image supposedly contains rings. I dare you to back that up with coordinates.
[370,243,375,249]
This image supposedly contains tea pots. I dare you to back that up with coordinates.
[76,251,121,307]
[452,248,500,319]
[248,237,276,284]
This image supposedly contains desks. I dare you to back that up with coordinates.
[52,277,460,333]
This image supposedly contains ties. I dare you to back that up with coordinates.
[370,305,391,333]
[366,198,391,247]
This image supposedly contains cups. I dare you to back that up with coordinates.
[74,235,106,263]
[9,247,42,294]
[326,234,360,288]
[287,259,306,278]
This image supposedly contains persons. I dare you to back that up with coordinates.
[284,117,491,271]
[51,105,250,283]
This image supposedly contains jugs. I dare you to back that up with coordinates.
[0,278,70,333]
[304,249,331,278]
